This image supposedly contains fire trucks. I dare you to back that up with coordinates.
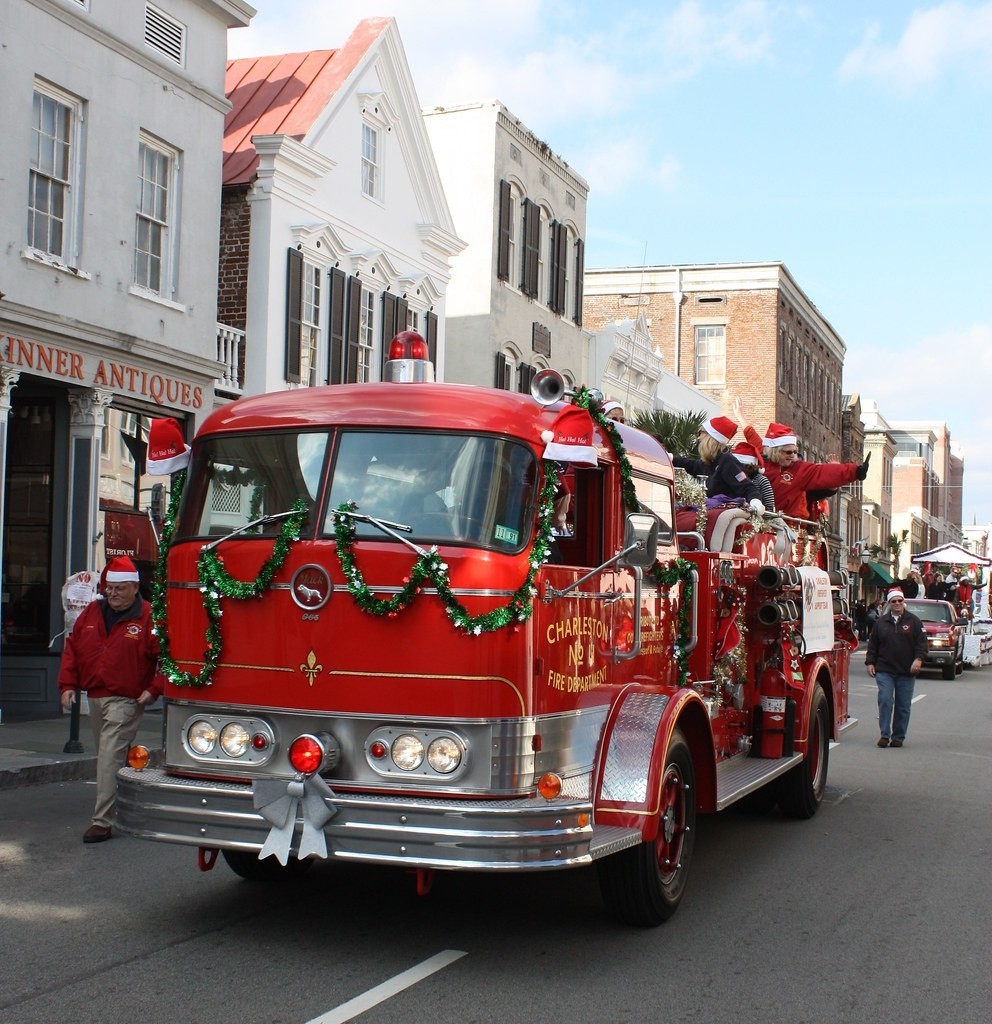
[98,325,859,930]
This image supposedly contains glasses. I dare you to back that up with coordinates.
[953,571,958,574]
[891,599,904,603]
[783,450,798,454]
[611,417,626,423]
[698,430,705,437]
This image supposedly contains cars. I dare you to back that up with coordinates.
[883,598,963,680]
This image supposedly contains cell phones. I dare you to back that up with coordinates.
[864,451,871,464]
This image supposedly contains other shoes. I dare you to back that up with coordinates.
[890,740,902,748]
[82,825,112,844]
[878,738,889,747]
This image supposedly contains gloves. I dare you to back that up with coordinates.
[858,451,872,482]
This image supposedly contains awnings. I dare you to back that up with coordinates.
[863,562,893,586]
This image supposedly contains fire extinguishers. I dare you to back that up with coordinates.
[757,642,788,758]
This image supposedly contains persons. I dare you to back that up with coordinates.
[57,555,168,843]
[667,416,766,517]
[549,470,572,536]
[763,423,871,530]
[729,442,776,520]
[600,399,624,424]
[731,396,841,533]
[855,564,988,748]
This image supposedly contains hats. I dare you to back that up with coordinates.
[763,423,797,447]
[959,575,969,581]
[602,399,623,416]
[481,443,512,473]
[541,408,601,470]
[887,587,907,608]
[702,416,737,444]
[146,418,192,476]
[729,442,765,474]
[99,555,140,595]
